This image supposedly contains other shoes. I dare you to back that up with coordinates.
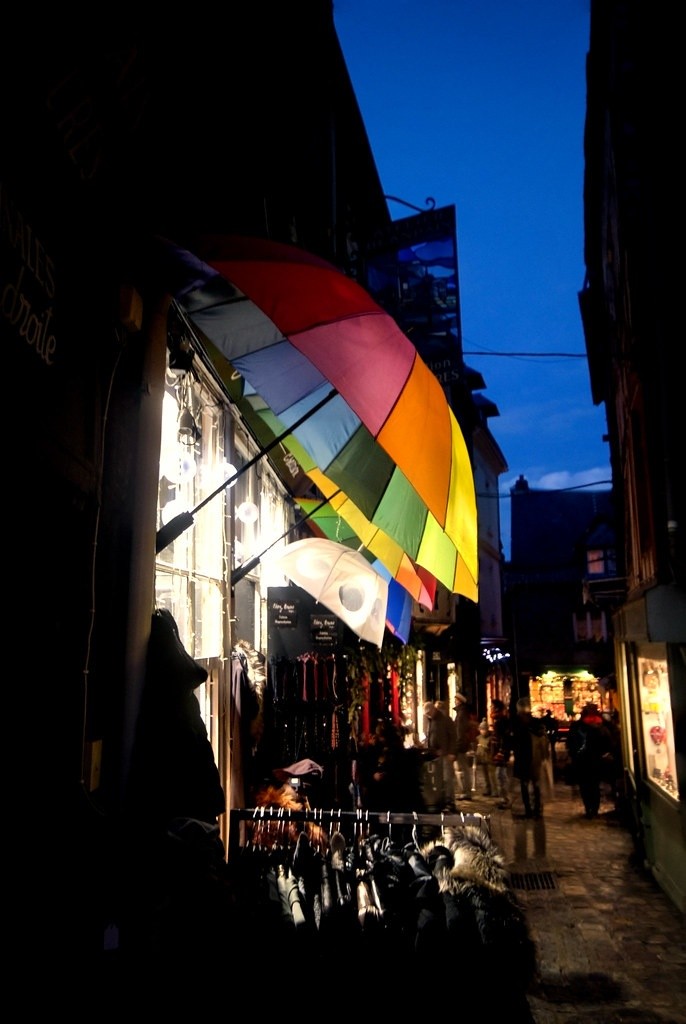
[456,794,470,801]
[586,810,598,817]
[517,812,532,818]
[483,790,491,796]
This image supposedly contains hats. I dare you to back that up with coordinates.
[478,718,488,729]
[454,690,469,703]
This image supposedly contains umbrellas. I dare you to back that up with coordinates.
[155,232,481,650]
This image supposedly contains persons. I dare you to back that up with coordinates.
[358,693,558,818]
[565,708,622,818]
[274,760,324,805]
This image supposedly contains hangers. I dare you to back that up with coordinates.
[459,811,466,830]
[440,813,445,838]
[251,806,371,857]
[412,812,421,851]
[386,811,393,842]
